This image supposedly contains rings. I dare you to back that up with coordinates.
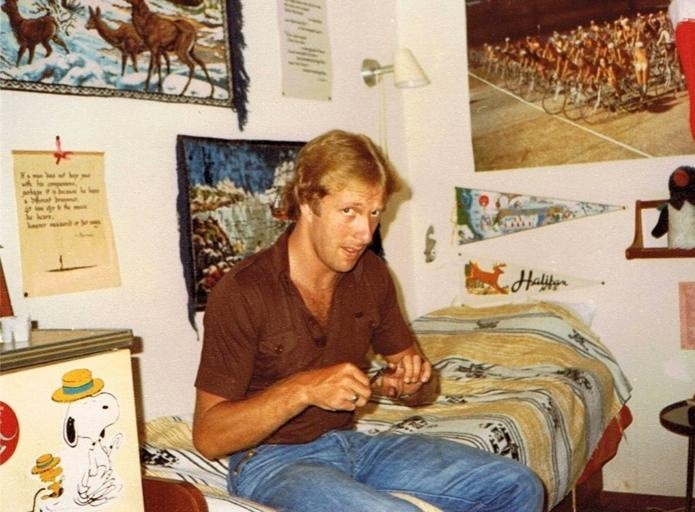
[352,395,359,404]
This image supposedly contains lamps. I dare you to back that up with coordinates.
[360,45,432,171]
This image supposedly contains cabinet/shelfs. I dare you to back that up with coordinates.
[0,327,145,511]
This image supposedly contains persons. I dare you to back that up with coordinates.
[477,10,675,106]
[193,128,547,512]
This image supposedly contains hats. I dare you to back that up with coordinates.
[51,367,104,404]
[31,453,61,475]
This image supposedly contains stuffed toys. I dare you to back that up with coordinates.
[651,164,695,250]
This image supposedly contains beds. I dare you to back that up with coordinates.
[136,301,632,510]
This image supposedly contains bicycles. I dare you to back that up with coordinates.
[474,16,688,126]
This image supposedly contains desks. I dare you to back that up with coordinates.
[661,398,695,511]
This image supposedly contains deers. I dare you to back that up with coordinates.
[1,0,69,67]
[84,5,171,77]
[125,0,215,99]
